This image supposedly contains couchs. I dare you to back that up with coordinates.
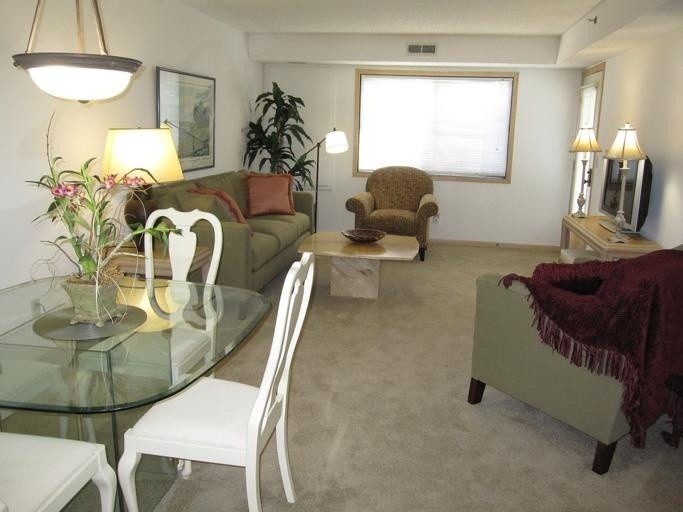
[125,170,316,292]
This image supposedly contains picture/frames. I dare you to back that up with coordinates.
[156,66,216,173]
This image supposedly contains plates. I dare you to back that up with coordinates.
[342,229,386,242]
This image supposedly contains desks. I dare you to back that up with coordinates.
[1,275,271,512]
[103,243,213,282]
[560,215,663,262]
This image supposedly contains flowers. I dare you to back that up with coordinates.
[26,110,183,327]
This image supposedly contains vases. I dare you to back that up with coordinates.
[60,279,123,327]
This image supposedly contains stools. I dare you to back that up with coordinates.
[560,248,600,265]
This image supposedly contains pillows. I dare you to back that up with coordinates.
[175,191,237,224]
[243,174,296,218]
[190,188,254,237]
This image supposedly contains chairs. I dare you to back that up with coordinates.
[346,166,438,261]
[117,252,316,512]
[0,431,118,512]
[467,245,683,475]
[77,208,225,470]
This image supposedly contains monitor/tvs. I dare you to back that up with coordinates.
[598,154,653,234]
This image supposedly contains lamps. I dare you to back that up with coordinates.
[302,128,348,233]
[568,128,602,218]
[100,128,184,253]
[603,123,647,244]
[116,275,181,333]
[12,0,142,104]
